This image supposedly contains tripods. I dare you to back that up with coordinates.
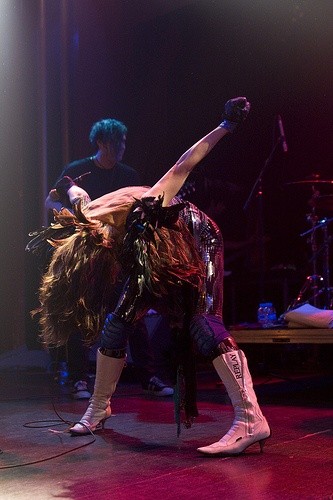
[278,186,333,324]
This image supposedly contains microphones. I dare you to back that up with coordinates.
[277,115,288,152]
[48,171,92,201]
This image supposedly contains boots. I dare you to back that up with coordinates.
[69,346,128,433]
[196,348,272,457]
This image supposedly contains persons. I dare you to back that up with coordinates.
[45,118,174,400]
[29,96,271,457]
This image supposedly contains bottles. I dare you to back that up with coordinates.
[58,362,67,384]
[258,303,276,323]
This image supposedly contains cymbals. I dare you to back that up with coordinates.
[264,180,333,228]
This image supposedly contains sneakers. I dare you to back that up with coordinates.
[72,380,92,399]
[149,375,174,395]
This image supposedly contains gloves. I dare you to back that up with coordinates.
[220,97,251,130]
[56,176,75,194]
